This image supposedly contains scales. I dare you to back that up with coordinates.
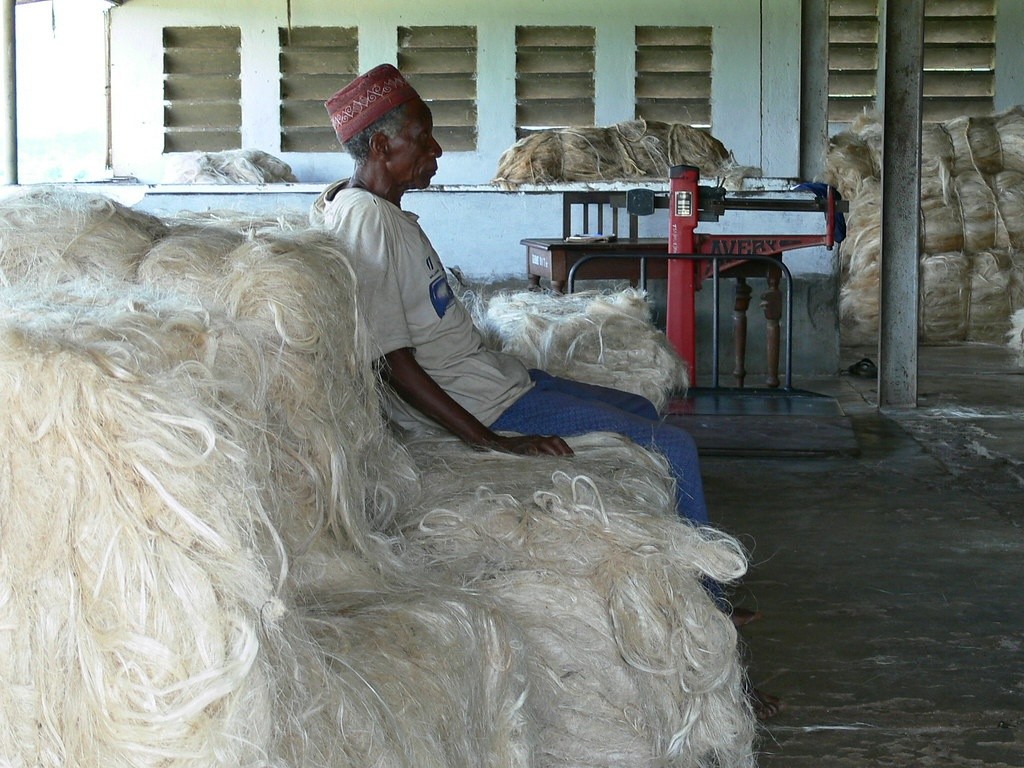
[607,162,864,461]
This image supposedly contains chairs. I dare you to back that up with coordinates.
[563,191,638,292]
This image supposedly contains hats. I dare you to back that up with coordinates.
[324,61,419,145]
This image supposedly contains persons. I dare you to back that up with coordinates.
[308,63,754,624]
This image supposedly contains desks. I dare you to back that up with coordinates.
[520,236,779,389]
